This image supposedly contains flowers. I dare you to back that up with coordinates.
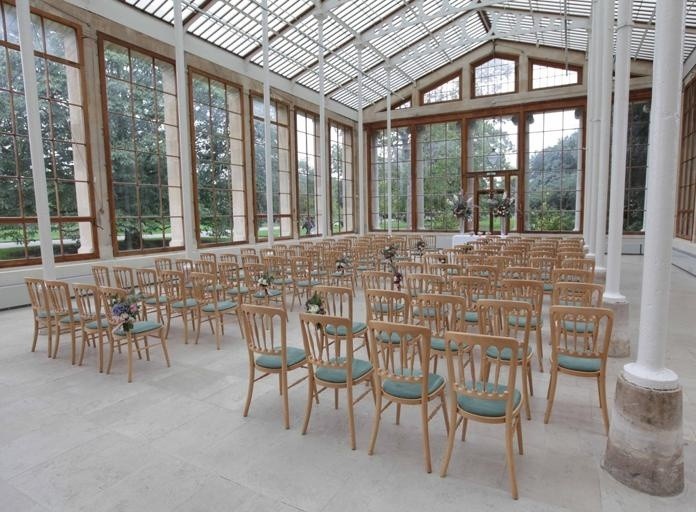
[417,241,425,256]
[334,256,348,271]
[380,244,399,263]
[463,243,472,253]
[439,256,446,264]
[255,272,275,296]
[306,291,327,330]
[109,299,141,335]
[393,273,402,290]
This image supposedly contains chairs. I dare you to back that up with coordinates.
[327,252,355,299]
[443,236,585,259]
[361,272,404,321]
[92,266,111,287]
[291,257,326,310]
[561,259,596,270]
[136,269,180,323]
[242,304,320,430]
[240,244,301,256]
[505,267,541,302]
[552,269,593,306]
[300,312,381,450]
[253,264,289,328]
[264,256,288,279]
[501,279,544,372]
[161,270,215,344]
[100,286,170,384]
[487,256,513,291]
[155,259,178,296]
[220,254,237,263]
[314,285,370,361]
[451,275,493,335]
[418,294,476,381]
[46,280,114,365]
[544,305,614,435]
[527,258,558,295]
[113,266,143,302]
[553,282,604,353]
[456,255,483,265]
[368,320,450,475]
[176,258,193,272]
[241,255,259,264]
[422,254,450,293]
[395,262,424,297]
[430,263,461,296]
[406,274,450,331]
[199,253,216,261]
[465,266,497,299]
[219,262,251,307]
[24,277,90,358]
[441,331,524,501]
[366,289,423,369]
[477,298,533,395]
[72,283,142,373]
[300,234,437,276]
[190,272,244,349]
[195,260,217,274]
